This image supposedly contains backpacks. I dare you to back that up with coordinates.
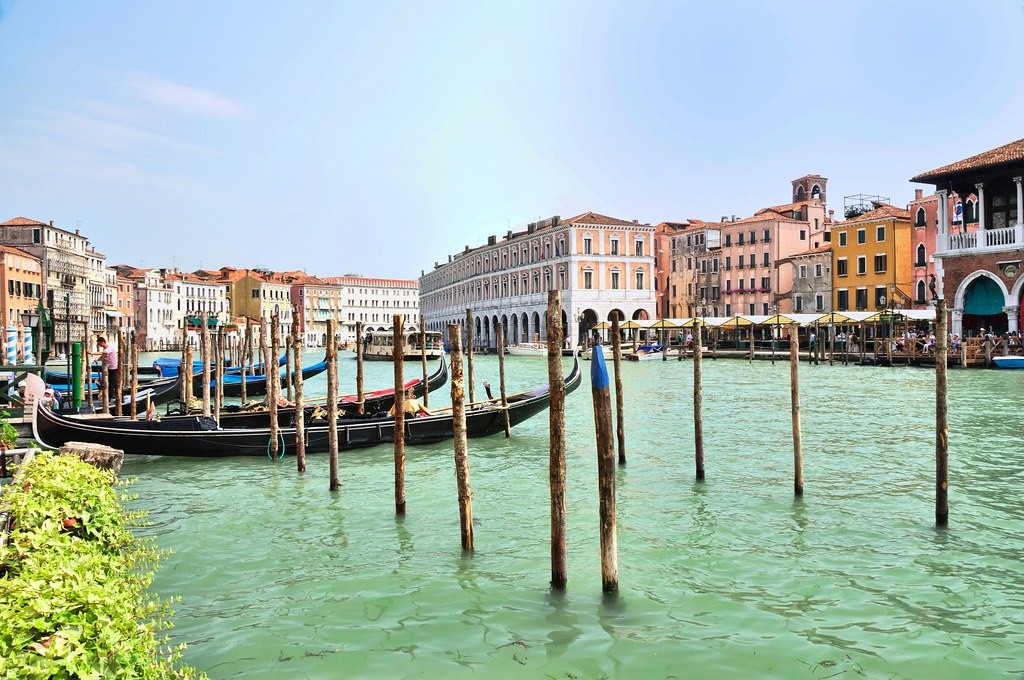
[54,391,63,410]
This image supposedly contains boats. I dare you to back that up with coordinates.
[363,330,445,361]
[0,348,585,452]
[505,342,681,361]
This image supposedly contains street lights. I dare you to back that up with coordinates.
[878,283,906,356]
[695,298,712,340]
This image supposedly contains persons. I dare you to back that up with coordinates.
[929,274,938,300]
[922,331,935,353]
[809,332,816,352]
[847,328,1024,353]
[687,331,693,350]
[95,337,125,406]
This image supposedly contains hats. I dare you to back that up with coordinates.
[18,381,26,387]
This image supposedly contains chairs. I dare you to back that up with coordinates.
[886,336,1005,356]
